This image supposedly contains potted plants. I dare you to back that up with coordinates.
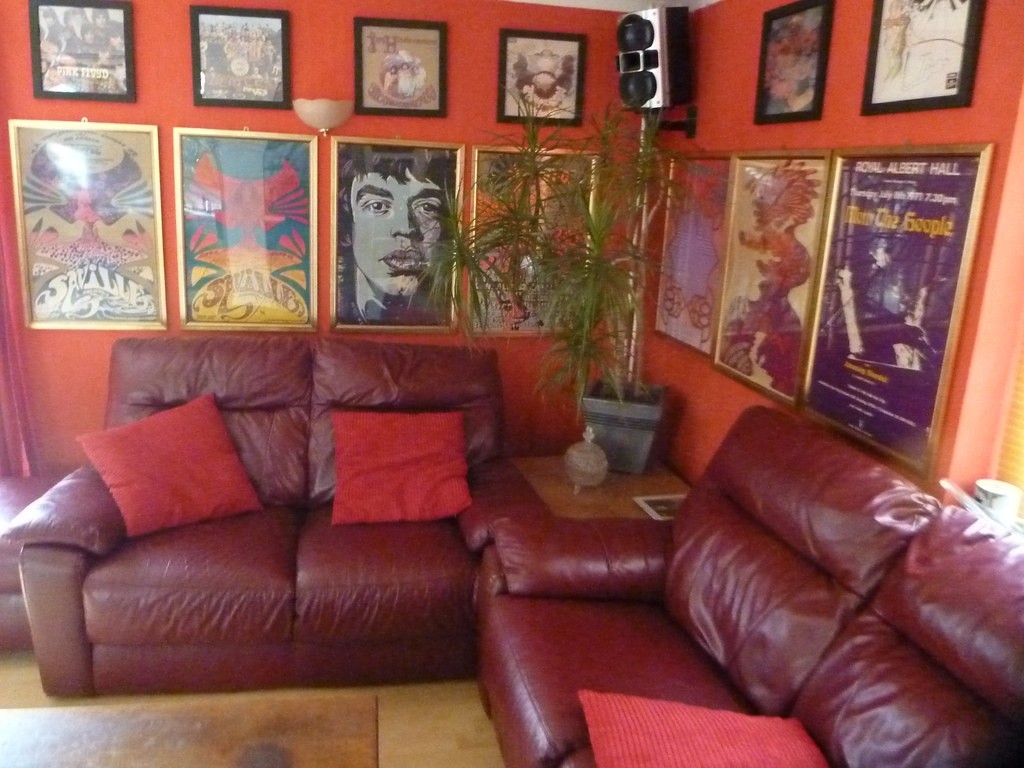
[418,96,705,478]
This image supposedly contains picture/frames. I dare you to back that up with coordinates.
[471,143,597,333]
[29,0,137,102]
[754,0,834,124]
[329,134,464,330]
[651,144,995,479]
[9,117,168,332]
[497,29,587,127]
[862,0,985,116]
[190,4,291,110]
[173,125,318,332]
[353,16,447,116]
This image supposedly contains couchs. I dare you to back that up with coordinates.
[1,335,544,701]
[477,402,1024,767]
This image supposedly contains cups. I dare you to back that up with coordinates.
[973,478,1023,527]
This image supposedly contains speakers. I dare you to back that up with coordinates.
[616,6,691,110]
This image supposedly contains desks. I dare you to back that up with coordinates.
[513,452,691,517]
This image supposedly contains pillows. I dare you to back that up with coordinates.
[329,406,473,525]
[579,689,829,767]
[74,392,266,535]
